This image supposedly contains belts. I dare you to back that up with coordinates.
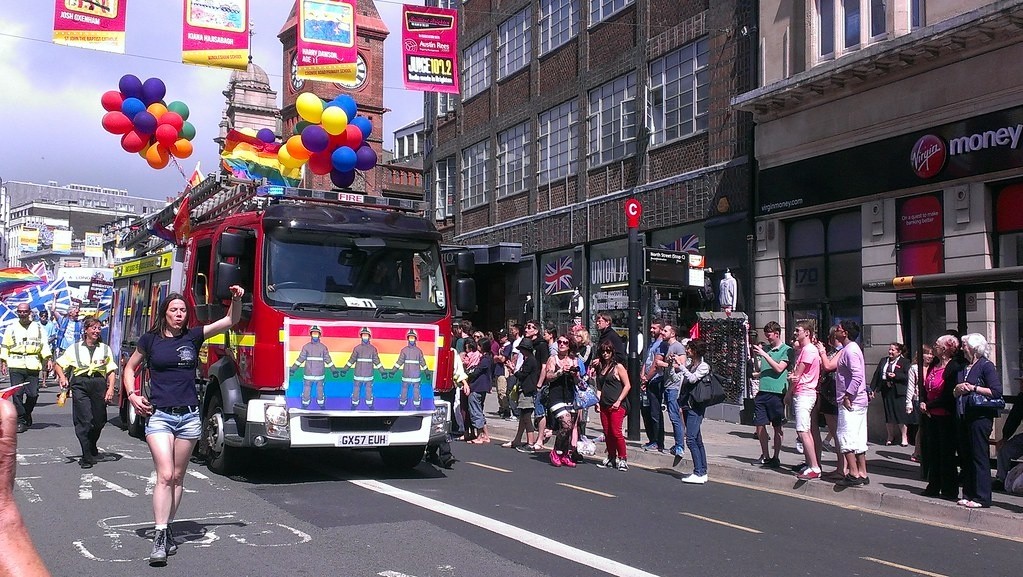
[159,405,195,416]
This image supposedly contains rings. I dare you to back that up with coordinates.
[961,390,962,391]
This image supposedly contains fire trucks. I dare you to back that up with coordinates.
[108,171,477,479]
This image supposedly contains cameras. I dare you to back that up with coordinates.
[565,366,580,376]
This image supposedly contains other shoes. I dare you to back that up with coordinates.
[16,409,32,434]
[81,443,99,469]
[56,393,70,398]
[750,431,1011,508]
[148,523,176,565]
[453,425,708,484]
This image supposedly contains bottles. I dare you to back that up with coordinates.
[640,392,643,405]
[642,391,650,406]
[582,408,588,421]
[57,388,68,407]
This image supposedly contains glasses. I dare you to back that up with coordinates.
[40,314,48,318]
[524,324,536,329]
[473,335,480,339]
[601,350,613,354]
[17,310,29,314]
[557,340,569,345]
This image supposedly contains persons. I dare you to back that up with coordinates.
[746,319,870,488]
[521,296,534,331]
[267,252,324,293]
[568,290,584,319]
[358,260,391,295]
[425,313,631,471]
[696,277,715,312]
[123,285,244,562]
[920,329,1003,508]
[869,343,934,462]
[639,318,708,484]
[718,273,737,310]
[0,397,50,577]
[0,291,118,469]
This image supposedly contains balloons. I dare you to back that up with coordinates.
[240,91,377,188]
[101,74,196,169]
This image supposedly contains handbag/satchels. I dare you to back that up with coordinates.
[969,362,1004,412]
[509,384,519,402]
[573,379,600,410]
[689,367,726,407]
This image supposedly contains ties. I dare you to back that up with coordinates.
[887,360,896,388]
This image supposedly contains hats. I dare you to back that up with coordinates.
[516,339,534,352]
[497,328,509,336]
[1013,373,1023,381]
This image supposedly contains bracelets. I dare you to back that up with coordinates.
[232,298,241,302]
[128,391,133,396]
[974,386,978,392]
[819,349,826,356]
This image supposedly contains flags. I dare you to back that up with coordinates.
[220,129,302,187]
[544,255,573,295]
[0,262,114,345]
[659,234,699,254]
